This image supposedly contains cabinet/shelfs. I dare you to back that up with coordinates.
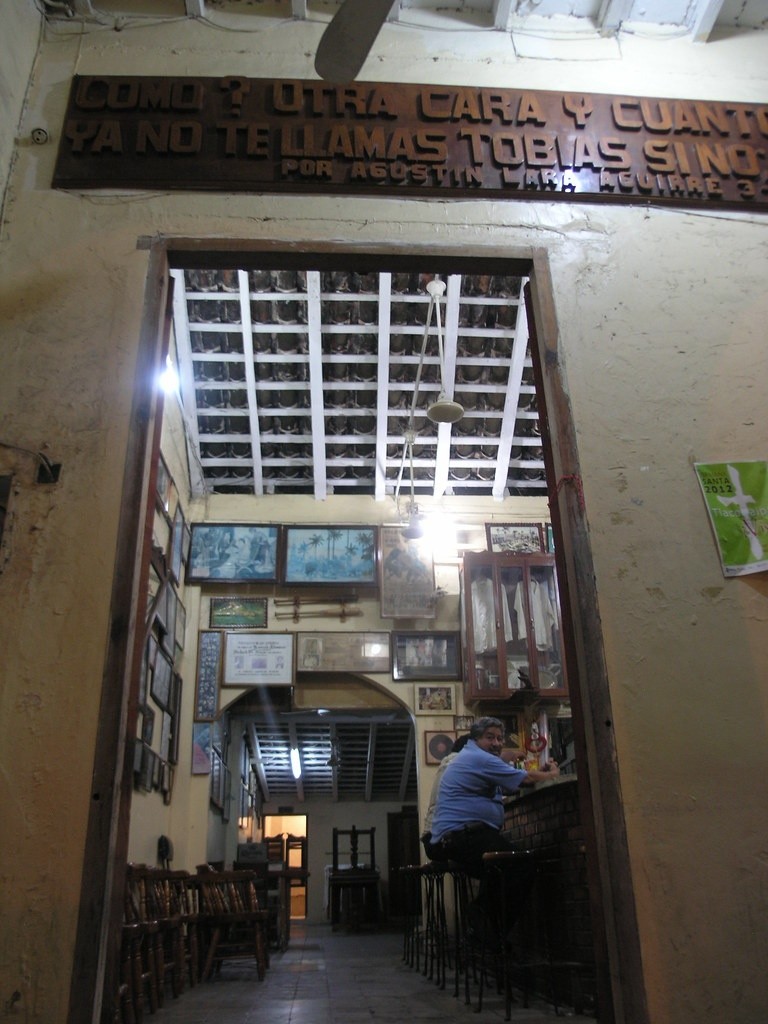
[458,552,569,707]
[328,824,381,933]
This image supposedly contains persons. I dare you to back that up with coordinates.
[428,718,561,953]
[421,732,494,931]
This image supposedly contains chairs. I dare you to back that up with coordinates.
[115,860,272,1024]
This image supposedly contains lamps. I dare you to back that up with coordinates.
[425,278,465,424]
[400,428,423,539]
[290,747,302,780]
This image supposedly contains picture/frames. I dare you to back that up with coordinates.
[453,716,475,730]
[378,523,436,619]
[208,596,268,628]
[414,682,457,716]
[193,629,225,722]
[132,448,191,808]
[424,730,457,765]
[192,711,262,830]
[185,521,283,584]
[479,709,527,757]
[456,730,471,739]
[484,522,545,554]
[391,630,461,681]
[433,562,461,595]
[295,631,392,673]
[545,523,556,555]
[280,524,381,586]
[221,630,296,686]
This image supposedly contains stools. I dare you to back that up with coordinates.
[399,851,586,1023]
